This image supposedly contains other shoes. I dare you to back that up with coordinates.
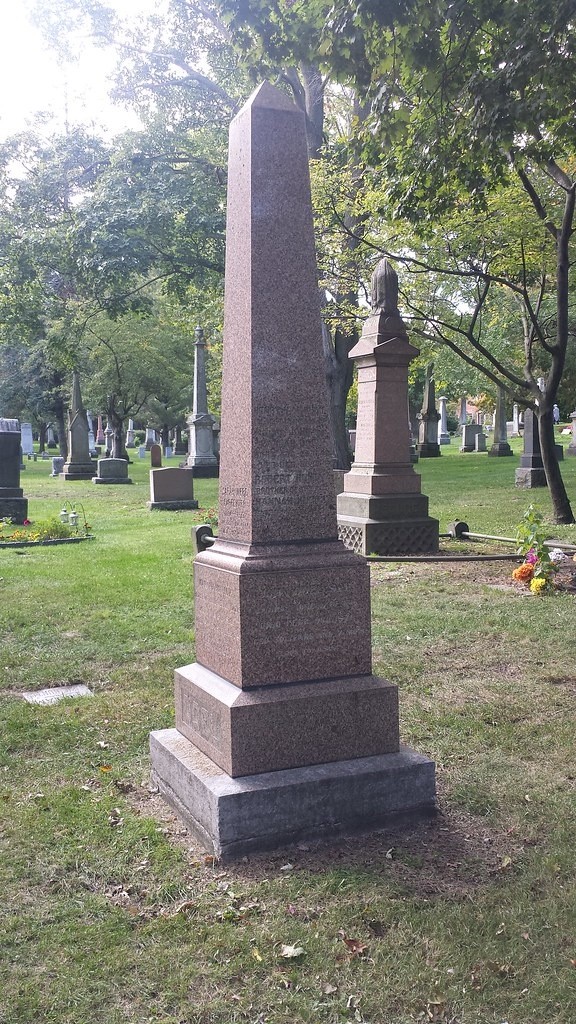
[553,423,555,424]
[557,423,559,424]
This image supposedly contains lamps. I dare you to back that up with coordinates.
[59,500,78,534]
[69,502,88,535]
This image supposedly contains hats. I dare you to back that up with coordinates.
[553,404,558,407]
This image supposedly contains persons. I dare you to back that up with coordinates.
[553,404,559,425]
